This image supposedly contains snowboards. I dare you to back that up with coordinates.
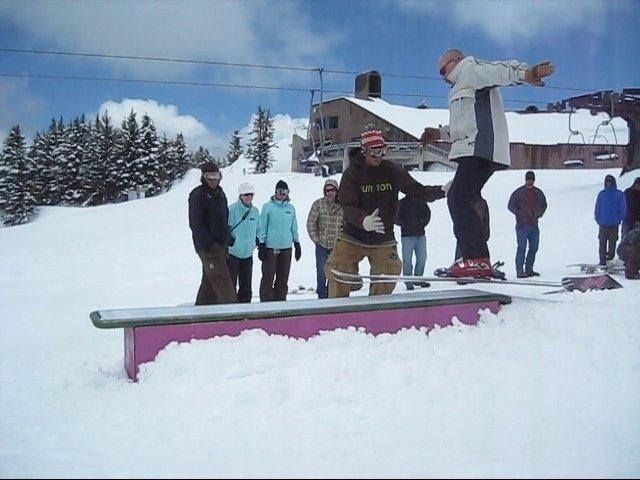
[563,274,623,291]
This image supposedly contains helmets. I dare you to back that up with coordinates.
[238,182,254,199]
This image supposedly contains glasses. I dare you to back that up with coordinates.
[440,60,453,75]
[326,188,335,192]
[204,171,221,181]
[369,149,387,158]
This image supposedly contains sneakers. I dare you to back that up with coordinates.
[447,258,493,278]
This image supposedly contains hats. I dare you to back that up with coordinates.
[360,123,386,153]
[276,180,288,189]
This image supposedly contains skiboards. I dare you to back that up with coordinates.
[565,260,626,274]
[330,269,574,287]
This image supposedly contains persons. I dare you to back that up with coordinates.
[325,123,454,298]
[188,162,239,305]
[257,181,302,302]
[228,183,260,303]
[617,225,640,279]
[307,179,344,299]
[622,177,640,240]
[419,49,554,277]
[594,175,626,265]
[507,171,547,278]
[398,196,431,291]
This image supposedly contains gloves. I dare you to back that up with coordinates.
[363,208,385,233]
[258,243,266,262]
[441,179,454,197]
[295,242,301,260]
